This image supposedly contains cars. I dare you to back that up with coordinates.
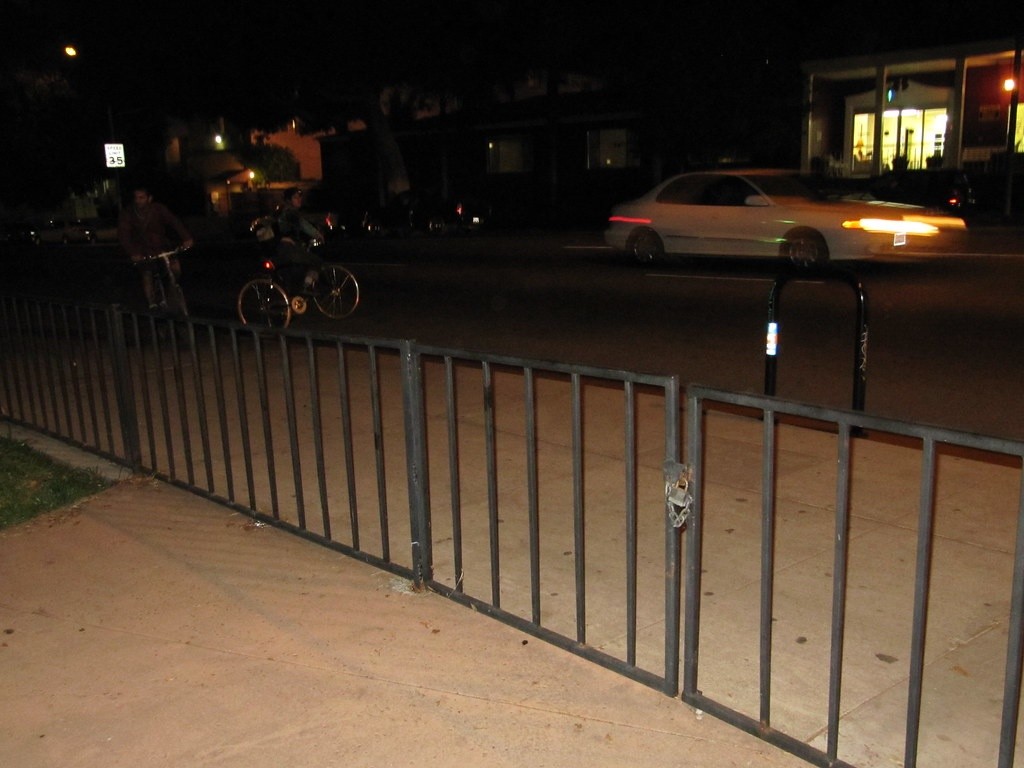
[362,187,492,237]
[39,218,97,244]
[226,187,362,259]
[603,167,972,279]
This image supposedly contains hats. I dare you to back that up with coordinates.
[283,188,305,205]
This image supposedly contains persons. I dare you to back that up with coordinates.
[271,187,325,300]
[117,188,193,311]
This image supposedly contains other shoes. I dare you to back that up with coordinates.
[149,302,160,314]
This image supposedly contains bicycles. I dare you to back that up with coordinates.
[136,243,193,350]
[235,236,360,340]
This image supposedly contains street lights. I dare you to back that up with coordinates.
[63,44,122,218]
